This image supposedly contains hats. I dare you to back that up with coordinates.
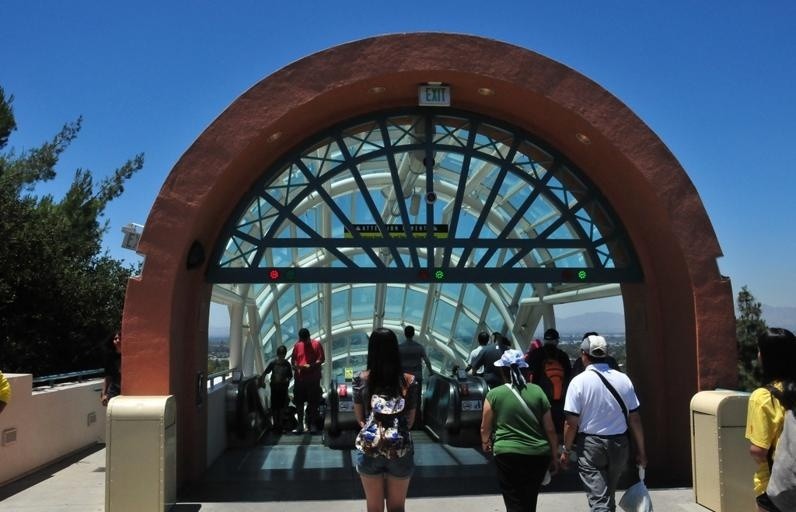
[544,328,560,340]
[579,335,608,359]
[493,348,529,368]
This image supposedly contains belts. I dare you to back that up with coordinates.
[585,432,626,440]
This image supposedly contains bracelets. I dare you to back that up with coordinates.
[563,444,571,455]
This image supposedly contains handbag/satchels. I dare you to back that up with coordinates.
[540,469,552,486]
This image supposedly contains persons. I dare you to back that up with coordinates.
[101,330,121,406]
[0,370,11,414]
[352,328,419,512]
[745,327,796,512]
[559,335,648,512]
[481,349,558,512]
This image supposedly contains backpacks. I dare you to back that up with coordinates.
[760,384,796,512]
[355,370,413,461]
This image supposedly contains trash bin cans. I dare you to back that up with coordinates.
[105,394,177,512]
[690,389,757,512]
